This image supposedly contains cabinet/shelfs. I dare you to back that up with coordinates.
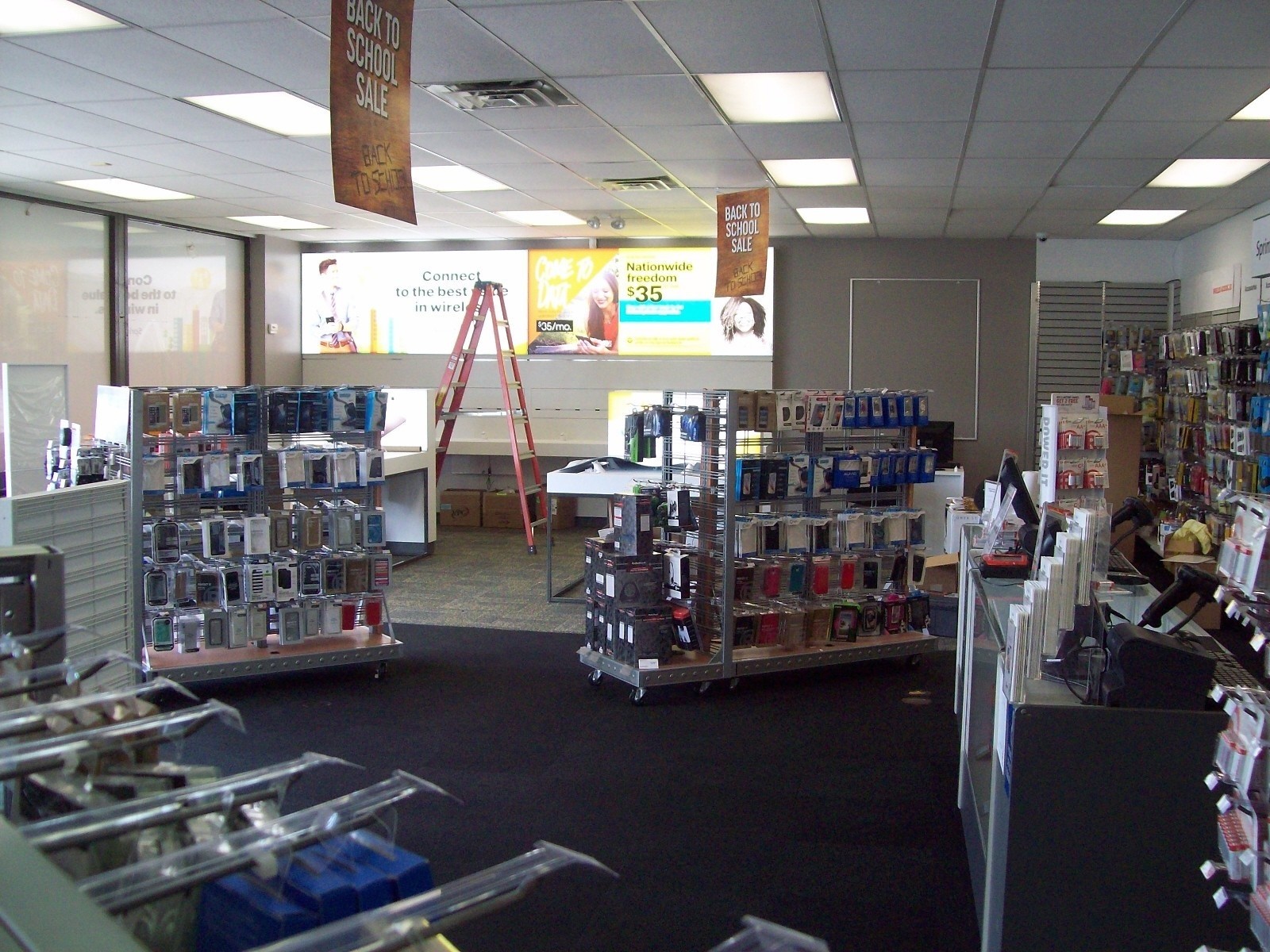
[132,388,403,683]
[703,384,922,651]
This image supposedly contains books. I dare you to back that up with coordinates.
[1002,507,1092,702]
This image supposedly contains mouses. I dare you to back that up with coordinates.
[1172,630,1201,643]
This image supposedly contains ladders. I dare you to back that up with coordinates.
[434,281,555,556]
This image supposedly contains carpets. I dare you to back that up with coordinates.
[152,622,984,952]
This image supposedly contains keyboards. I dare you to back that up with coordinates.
[941,463,960,468]
[1109,545,1131,573]
[1207,650,1262,698]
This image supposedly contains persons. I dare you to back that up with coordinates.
[314,259,351,335]
[577,273,619,354]
[719,296,768,356]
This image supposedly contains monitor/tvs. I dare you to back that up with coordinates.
[1041,587,1109,677]
[1000,457,1040,525]
[916,421,954,470]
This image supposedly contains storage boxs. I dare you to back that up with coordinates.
[928,595,983,638]
[439,489,482,529]
[925,553,961,593]
[535,492,575,531]
[482,487,530,532]
[585,493,671,667]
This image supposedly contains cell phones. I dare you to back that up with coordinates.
[326,316,334,323]
[836,604,903,640]
[576,334,593,346]
[146,403,387,432]
[738,405,843,496]
